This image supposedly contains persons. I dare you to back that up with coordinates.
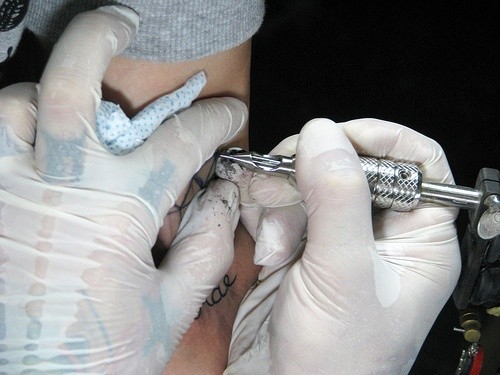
[0,82,462,375]
[0,0,267,375]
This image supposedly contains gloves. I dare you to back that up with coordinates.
[1,5,250,372]
[226,115,462,374]
